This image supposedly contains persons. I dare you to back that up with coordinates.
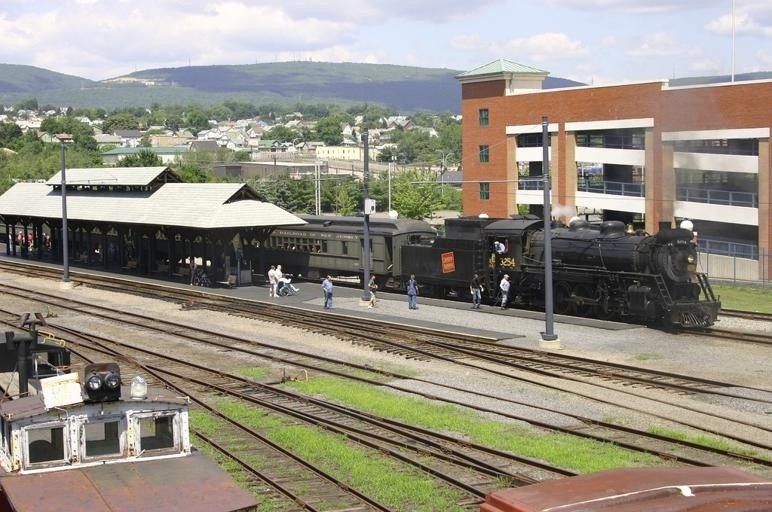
[499,274,512,310]
[7,229,52,255]
[469,273,485,309]
[275,264,283,294]
[200,270,208,283]
[277,273,299,296]
[267,264,280,298]
[406,273,419,310]
[321,275,335,310]
[367,273,379,310]
[189,258,200,286]
[493,241,506,272]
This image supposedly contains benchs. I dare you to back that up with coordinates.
[216,274,237,289]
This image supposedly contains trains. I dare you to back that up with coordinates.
[0,312,263,512]
[0,213,723,336]
[479,464,772,512]
